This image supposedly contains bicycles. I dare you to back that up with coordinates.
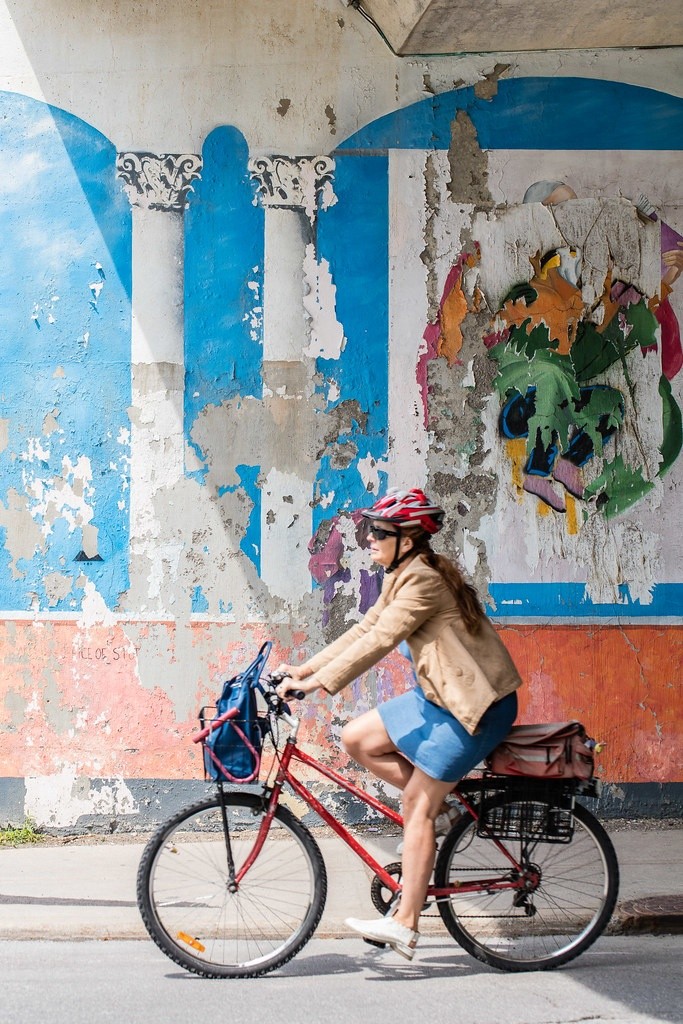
[135,673,621,980]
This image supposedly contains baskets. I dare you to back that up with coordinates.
[198,706,269,784]
[476,773,575,843]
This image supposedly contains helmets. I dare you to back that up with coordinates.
[361,490,444,534]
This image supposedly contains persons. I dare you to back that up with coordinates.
[270,488,522,963]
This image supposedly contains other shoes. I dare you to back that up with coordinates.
[347,916,419,960]
[396,807,460,855]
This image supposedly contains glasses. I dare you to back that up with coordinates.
[369,525,409,540]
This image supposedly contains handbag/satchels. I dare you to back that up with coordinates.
[486,723,593,779]
[205,642,273,782]
